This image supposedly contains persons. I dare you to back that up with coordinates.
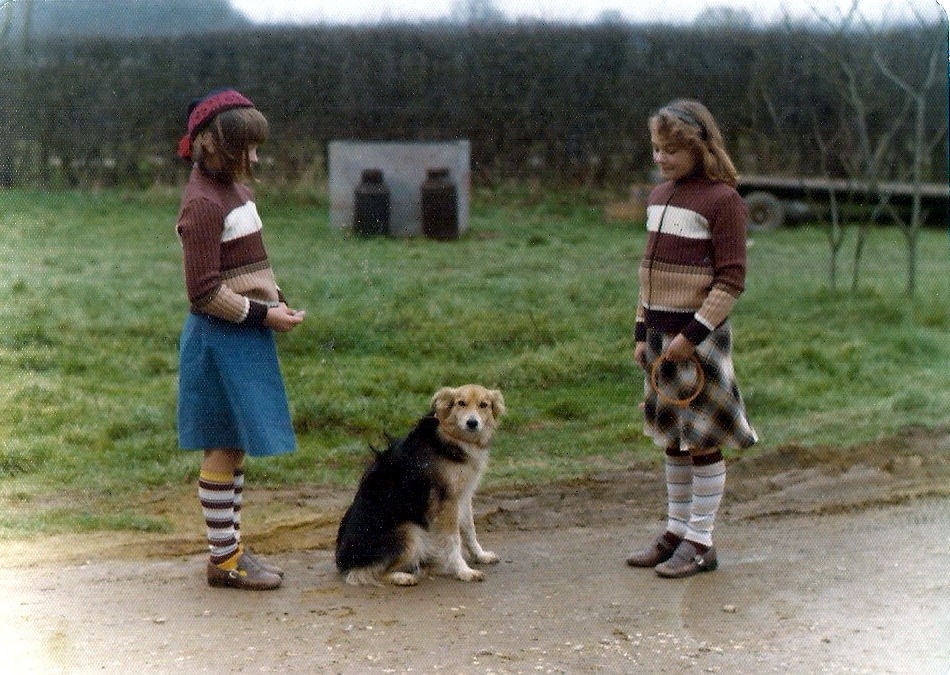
[176,89,306,591]
[625,101,758,580]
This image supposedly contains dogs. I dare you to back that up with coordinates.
[336,381,505,591]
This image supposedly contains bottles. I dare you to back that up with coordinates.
[354,170,389,236]
[421,168,458,239]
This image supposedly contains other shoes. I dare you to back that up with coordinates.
[655,540,718,578]
[207,550,284,590]
[628,536,681,567]
